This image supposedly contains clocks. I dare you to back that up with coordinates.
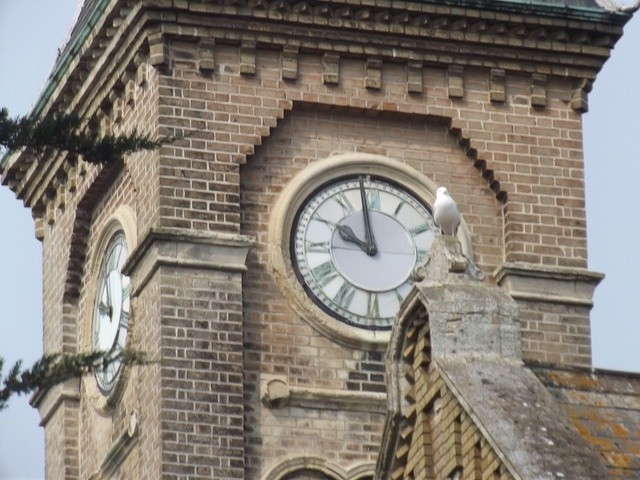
[289,172,443,333]
[85,229,132,395]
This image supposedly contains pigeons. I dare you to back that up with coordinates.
[434,186,460,236]
